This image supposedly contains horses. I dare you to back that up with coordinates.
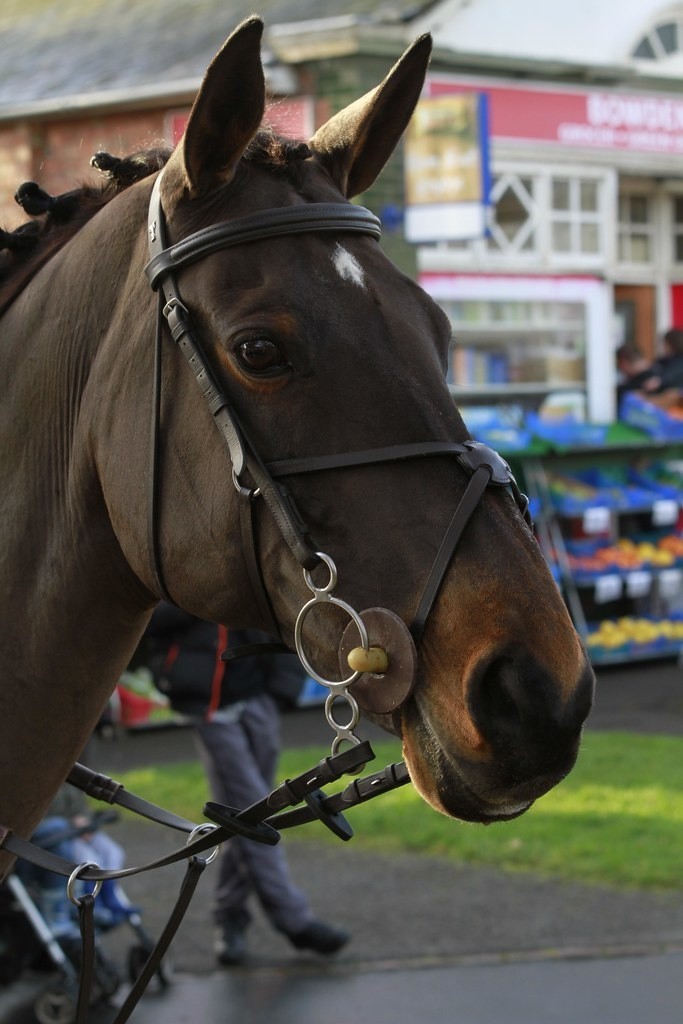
[0,12,599,887]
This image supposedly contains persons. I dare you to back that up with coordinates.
[26,783,124,933]
[129,602,349,967]
[614,329,683,407]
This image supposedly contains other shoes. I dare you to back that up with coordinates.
[214,921,247,966]
[288,918,351,957]
